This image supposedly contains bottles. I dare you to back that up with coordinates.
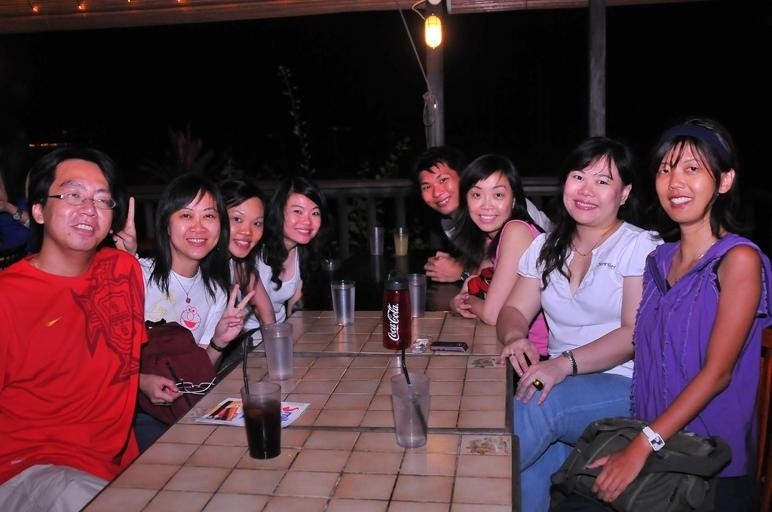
[382,271,413,348]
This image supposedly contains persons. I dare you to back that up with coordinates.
[450,154,551,393]
[551,117,770,510]
[206,178,276,380]
[0,169,33,254]
[497,136,666,510]
[412,147,482,283]
[240,175,333,352]
[1,148,149,512]
[110,170,257,453]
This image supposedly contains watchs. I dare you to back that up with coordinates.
[641,426,666,452]
[12,208,24,222]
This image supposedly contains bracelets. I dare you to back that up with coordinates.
[564,350,577,376]
[209,338,226,354]
[460,266,472,281]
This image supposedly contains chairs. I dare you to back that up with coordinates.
[757,323,772,510]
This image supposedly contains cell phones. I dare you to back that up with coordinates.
[430,341,467,352]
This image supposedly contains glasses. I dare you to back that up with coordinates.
[46,192,116,210]
[171,377,216,397]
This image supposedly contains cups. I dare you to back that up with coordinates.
[368,225,387,257]
[393,226,411,256]
[329,279,358,326]
[239,381,283,460]
[391,370,433,446]
[406,273,427,316]
[259,321,296,381]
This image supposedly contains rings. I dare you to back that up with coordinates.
[533,379,544,391]
[507,353,516,358]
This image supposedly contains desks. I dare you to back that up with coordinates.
[74,428,525,512]
[177,350,518,432]
[251,308,509,354]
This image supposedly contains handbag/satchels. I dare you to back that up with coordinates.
[137,319,218,426]
[550,417,731,511]
[218,327,251,378]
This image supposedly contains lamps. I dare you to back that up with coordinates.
[409,0,447,50]
